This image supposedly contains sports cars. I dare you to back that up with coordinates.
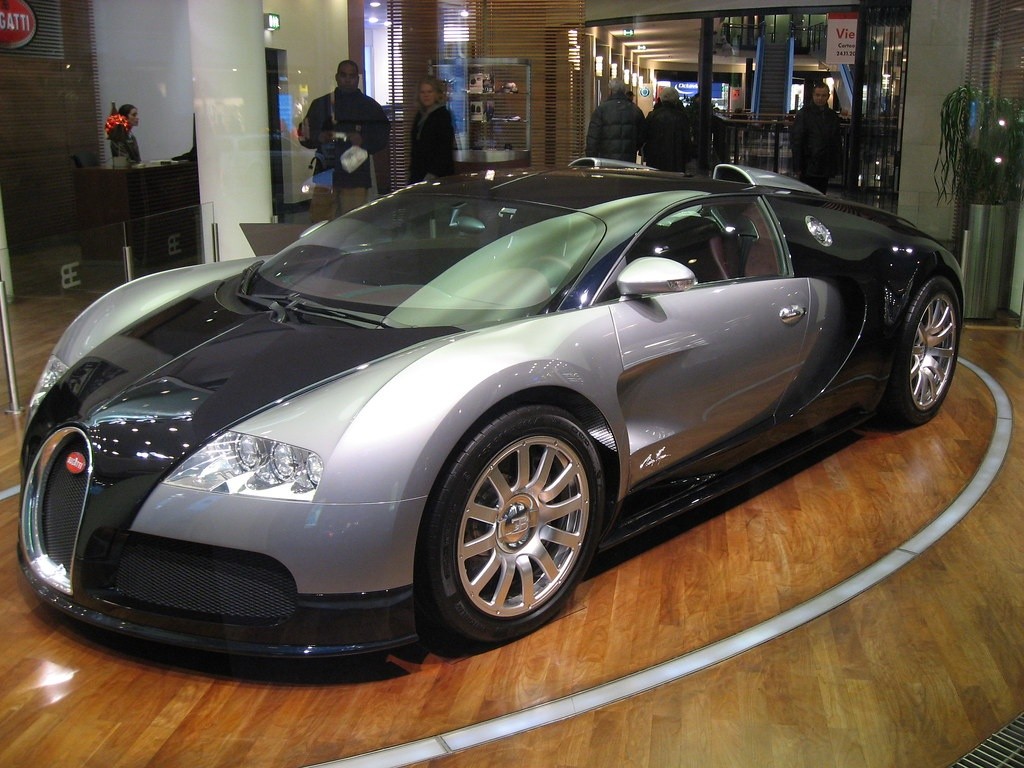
[19,159,965,663]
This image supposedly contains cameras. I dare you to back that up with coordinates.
[330,132,348,143]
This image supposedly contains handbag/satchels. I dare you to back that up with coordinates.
[340,145,368,174]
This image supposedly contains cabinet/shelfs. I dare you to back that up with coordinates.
[427,56,531,164]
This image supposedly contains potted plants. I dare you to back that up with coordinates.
[933,82,1024,320]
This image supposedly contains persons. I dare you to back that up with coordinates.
[110,104,142,163]
[585,77,729,176]
[409,76,456,184]
[298,61,392,224]
[790,83,841,195]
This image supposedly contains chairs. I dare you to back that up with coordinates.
[660,217,733,282]
[441,201,508,245]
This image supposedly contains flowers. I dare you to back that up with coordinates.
[105,114,131,156]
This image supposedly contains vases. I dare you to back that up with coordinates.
[112,157,131,169]
